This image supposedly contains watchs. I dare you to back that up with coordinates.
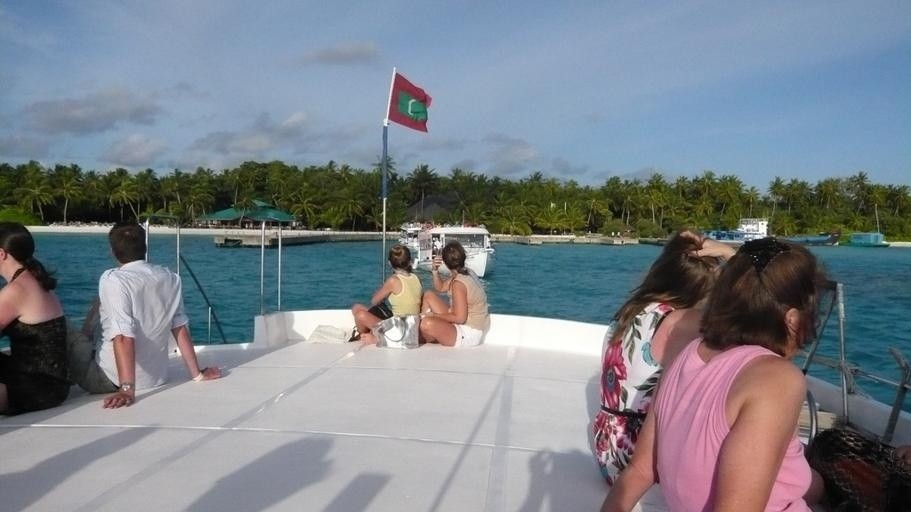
[116,383,136,391]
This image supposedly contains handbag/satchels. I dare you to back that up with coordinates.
[373,314,421,349]
[803,427,901,512]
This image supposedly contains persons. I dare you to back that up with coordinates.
[1,219,73,417]
[595,237,833,511]
[589,230,736,489]
[59,222,221,410]
[420,239,492,347]
[352,245,421,343]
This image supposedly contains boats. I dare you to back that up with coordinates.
[396,218,496,279]
[636,215,841,246]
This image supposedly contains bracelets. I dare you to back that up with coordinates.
[389,74,434,135]
[193,373,204,383]
[431,269,440,276]
